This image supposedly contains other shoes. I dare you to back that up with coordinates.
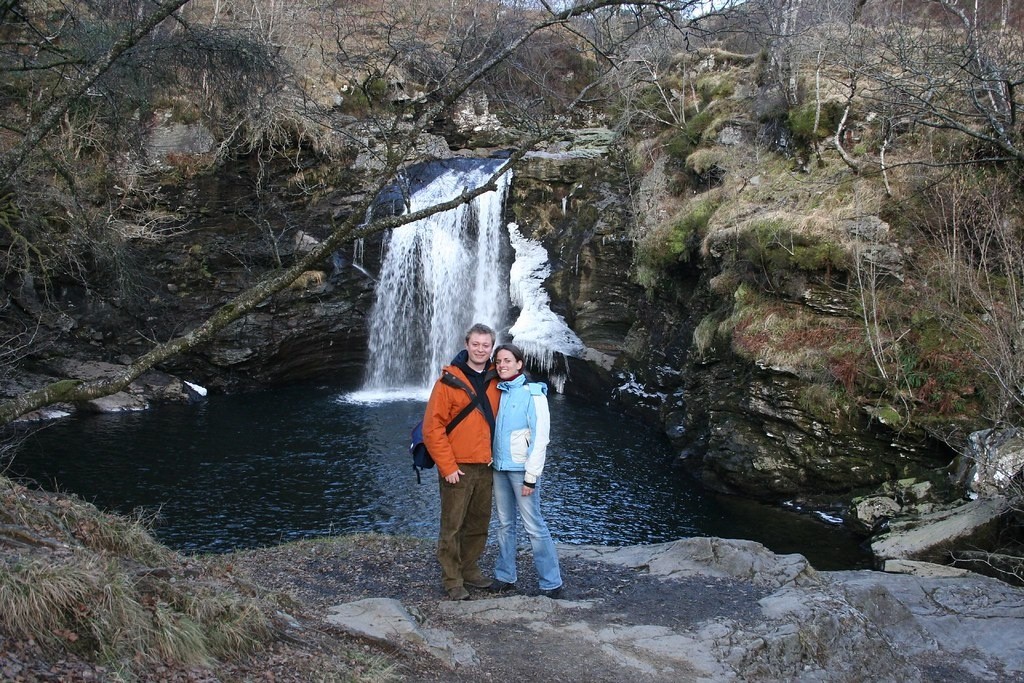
[447,586,470,600]
[463,575,494,587]
[542,585,562,596]
[489,579,514,593]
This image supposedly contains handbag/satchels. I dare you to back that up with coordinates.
[410,421,435,484]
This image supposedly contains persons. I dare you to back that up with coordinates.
[488,344,563,599]
[422,324,502,600]
